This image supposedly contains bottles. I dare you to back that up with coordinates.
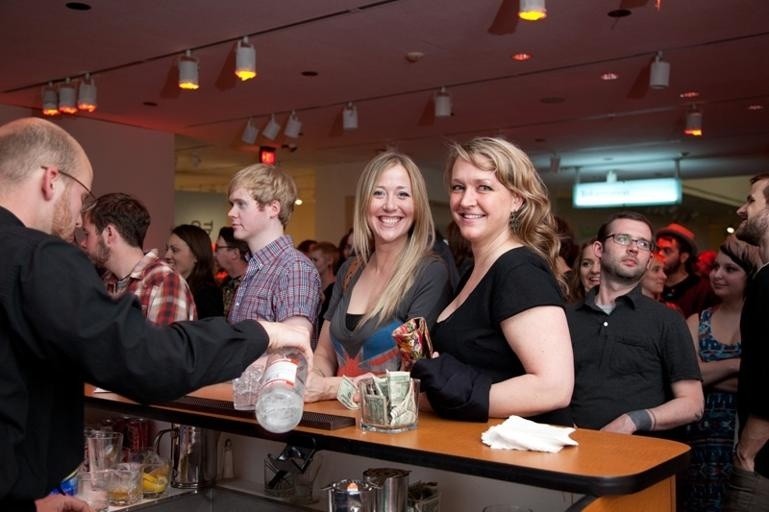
[255,347,309,433]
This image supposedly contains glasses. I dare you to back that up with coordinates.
[602,232,654,251]
[215,244,231,252]
[39,165,98,213]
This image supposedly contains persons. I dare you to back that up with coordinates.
[555,252,579,300]
[683,237,752,512]
[166,226,225,320]
[414,136,577,415]
[308,149,445,402]
[336,229,356,274]
[214,226,249,310]
[0,115,315,512]
[573,237,604,295]
[548,213,704,435]
[82,192,199,327]
[733,174,768,512]
[228,162,315,357]
[649,224,716,315]
[637,257,668,298]
[310,243,340,339]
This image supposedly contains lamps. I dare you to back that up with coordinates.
[685,112,702,137]
[517,1,547,23]
[342,105,358,130]
[571,177,683,208]
[76,74,97,111]
[262,115,280,141]
[42,82,58,115]
[648,52,670,90]
[240,117,259,145]
[176,51,199,90]
[234,37,256,82]
[57,77,78,114]
[432,86,452,117]
[283,114,302,139]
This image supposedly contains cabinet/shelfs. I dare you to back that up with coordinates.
[78,383,692,512]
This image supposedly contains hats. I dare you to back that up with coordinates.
[655,223,695,251]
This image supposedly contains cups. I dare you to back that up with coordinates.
[107,468,139,506]
[153,423,221,488]
[360,377,419,433]
[78,472,108,511]
[144,463,170,498]
[232,356,266,410]
[87,432,127,486]
[263,458,295,496]
